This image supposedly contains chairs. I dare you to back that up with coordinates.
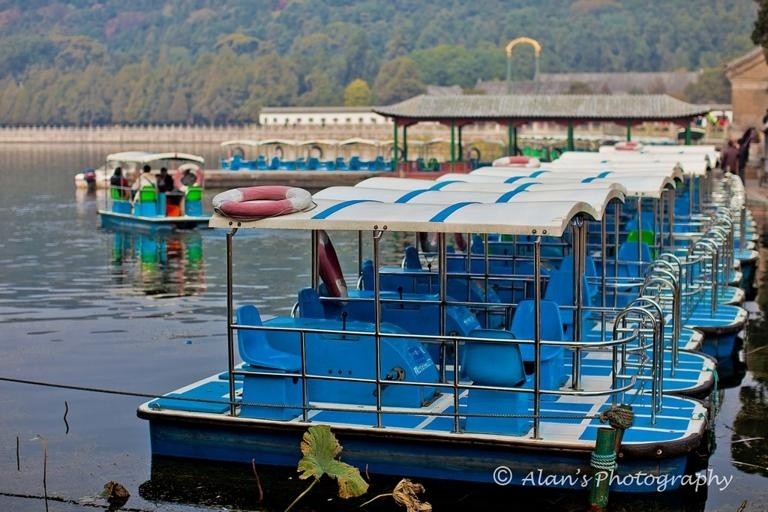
[184,187,203,216]
[111,231,202,270]
[604,166,701,291]
[362,259,382,290]
[234,303,314,422]
[133,184,157,217]
[297,287,325,318]
[404,246,422,269]
[220,155,477,170]
[520,145,561,162]
[458,255,599,437]
[109,185,131,214]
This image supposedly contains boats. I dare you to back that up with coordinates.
[73,136,762,512]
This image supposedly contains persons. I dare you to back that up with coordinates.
[126,162,142,185]
[156,167,173,192]
[109,167,128,198]
[130,164,157,202]
[180,168,197,186]
[715,109,768,188]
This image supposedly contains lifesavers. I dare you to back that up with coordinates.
[493,155,541,169]
[173,163,204,193]
[388,146,403,161]
[454,233,473,251]
[467,146,481,161]
[213,185,313,218]
[615,142,642,150]
[420,232,439,257]
[316,230,349,297]
[229,147,245,159]
[274,146,284,159]
[311,146,324,159]
